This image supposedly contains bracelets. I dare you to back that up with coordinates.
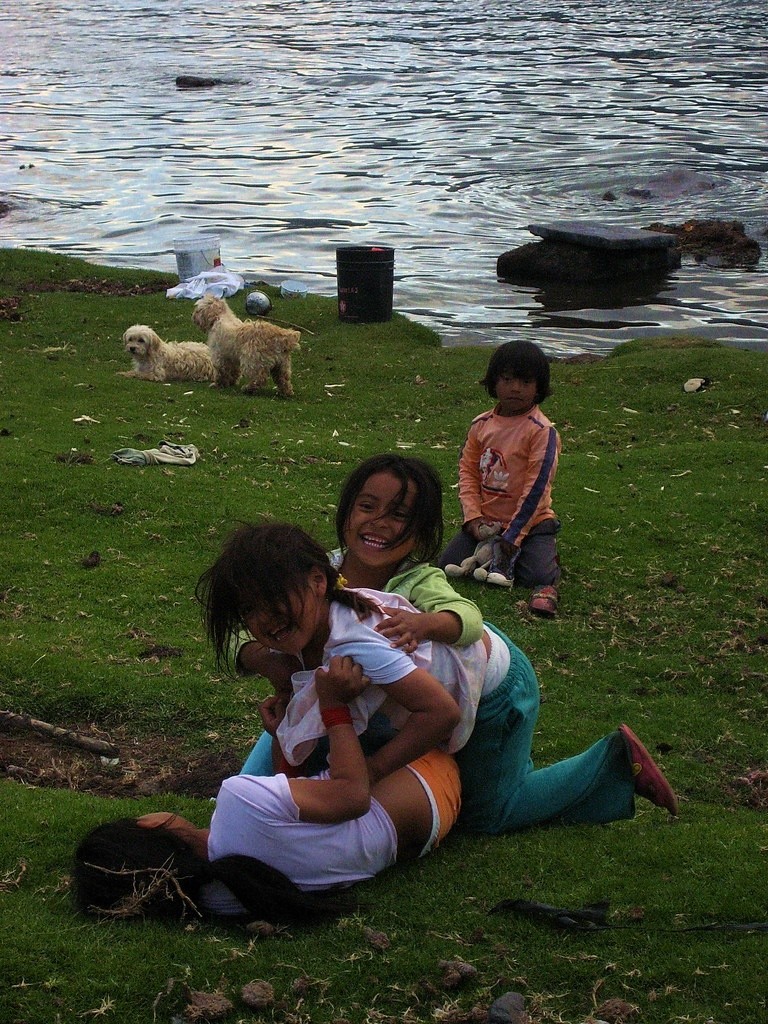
[320,704,353,731]
[277,755,305,777]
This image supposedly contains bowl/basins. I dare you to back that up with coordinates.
[281,280,307,300]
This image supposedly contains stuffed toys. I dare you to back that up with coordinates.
[442,520,503,582]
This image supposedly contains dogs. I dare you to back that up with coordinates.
[190,294,302,398]
[116,323,215,383]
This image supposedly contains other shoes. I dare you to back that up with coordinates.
[487,540,522,587]
[616,722,679,816]
[529,584,561,614]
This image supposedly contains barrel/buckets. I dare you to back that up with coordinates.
[173,234,222,282]
[337,246,393,323]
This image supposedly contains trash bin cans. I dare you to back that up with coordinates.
[334,243,397,325]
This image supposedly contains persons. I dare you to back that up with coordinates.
[438,339,561,619]
[73,654,464,938]
[192,450,682,834]
